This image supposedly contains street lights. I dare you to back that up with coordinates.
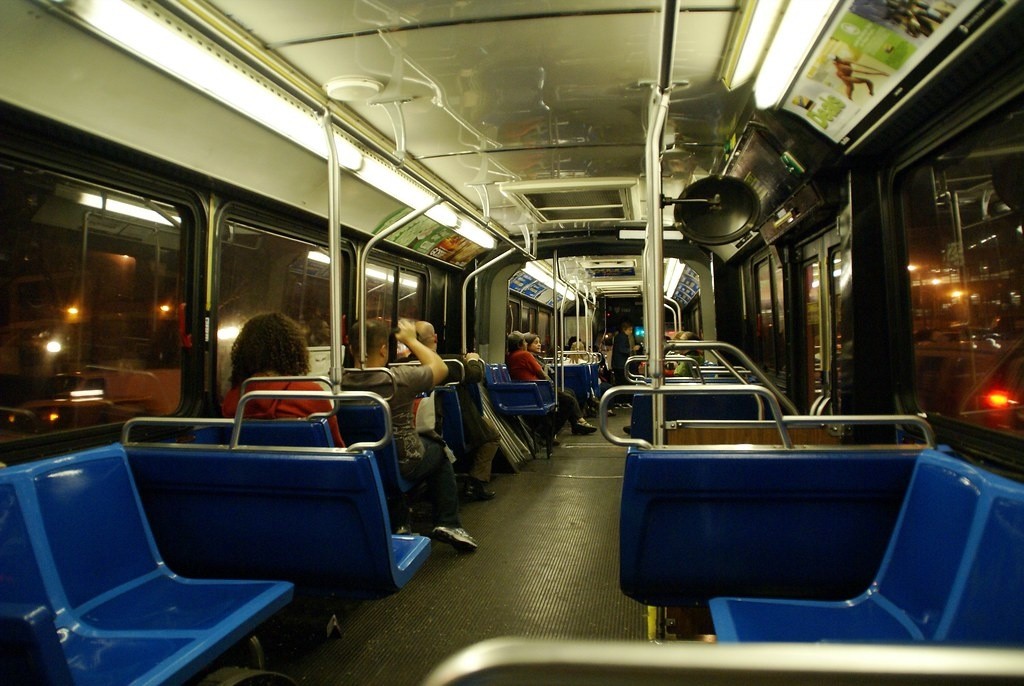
[906,264,926,332]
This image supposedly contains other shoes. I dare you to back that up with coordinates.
[608,412,616,416]
[624,426,631,433]
[465,485,496,498]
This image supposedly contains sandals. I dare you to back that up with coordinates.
[572,420,597,434]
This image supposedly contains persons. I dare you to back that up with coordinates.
[221,313,345,448]
[623,331,704,434]
[506,331,616,448]
[393,320,500,501]
[341,319,478,553]
[612,322,640,408]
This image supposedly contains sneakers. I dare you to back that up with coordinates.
[398,526,411,535]
[433,526,478,551]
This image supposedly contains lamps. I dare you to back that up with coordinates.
[663,258,685,298]
[521,261,575,301]
[52,0,500,252]
[717,0,841,108]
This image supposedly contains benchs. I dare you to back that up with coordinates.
[0,359,465,686]
[432,352,1024,686]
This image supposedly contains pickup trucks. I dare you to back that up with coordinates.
[9,371,154,431]
